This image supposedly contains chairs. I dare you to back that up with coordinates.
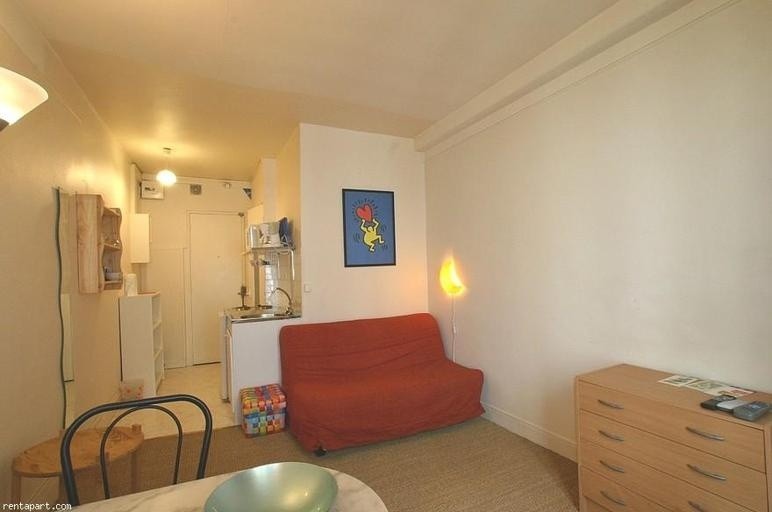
[60,394,213,510]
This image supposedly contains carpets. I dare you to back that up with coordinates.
[49,422,579,511]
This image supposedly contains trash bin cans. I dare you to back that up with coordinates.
[119,379,144,410]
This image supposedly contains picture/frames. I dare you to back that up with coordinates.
[340,187,397,268]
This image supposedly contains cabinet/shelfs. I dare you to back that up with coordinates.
[224,316,300,426]
[572,362,772,512]
[115,291,166,401]
[73,190,122,294]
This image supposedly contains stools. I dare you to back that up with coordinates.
[241,383,287,439]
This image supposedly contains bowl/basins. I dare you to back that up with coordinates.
[205,460,339,512]
[105,272,121,281]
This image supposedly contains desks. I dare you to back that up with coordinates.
[51,458,387,511]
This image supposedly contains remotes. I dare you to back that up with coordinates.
[700,392,771,422]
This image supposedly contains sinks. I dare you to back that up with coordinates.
[239,313,294,318]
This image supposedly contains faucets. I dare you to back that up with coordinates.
[270,287,293,315]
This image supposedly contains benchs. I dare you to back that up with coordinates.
[274,312,484,456]
